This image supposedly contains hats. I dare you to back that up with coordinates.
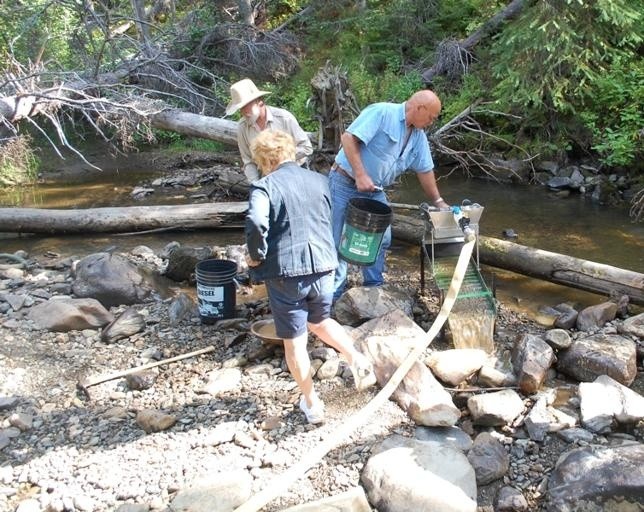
[225,79,272,116]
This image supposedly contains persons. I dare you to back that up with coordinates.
[244,128,376,424]
[328,90,451,308]
[225,78,313,184]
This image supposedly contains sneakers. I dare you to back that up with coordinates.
[300,399,325,424]
[349,354,377,391]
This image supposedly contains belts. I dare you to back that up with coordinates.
[333,161,348,176]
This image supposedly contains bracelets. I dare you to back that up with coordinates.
[433,197,444,205]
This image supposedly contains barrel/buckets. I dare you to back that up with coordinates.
[338,198,393,266]
[195,259,237,324]
